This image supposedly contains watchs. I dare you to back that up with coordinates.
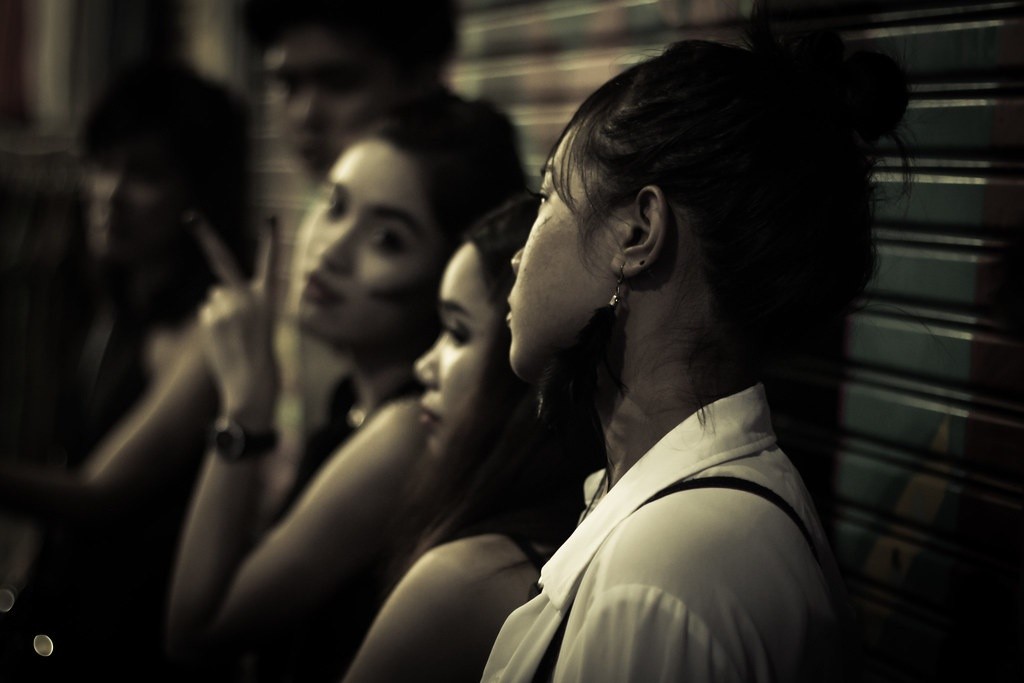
[207,416,280,465]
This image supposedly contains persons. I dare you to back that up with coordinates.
[234,0,465,192]
[2,61,256,607]
[337,196,604,683]
[482,0,922,683]
[24,102,524,683]
